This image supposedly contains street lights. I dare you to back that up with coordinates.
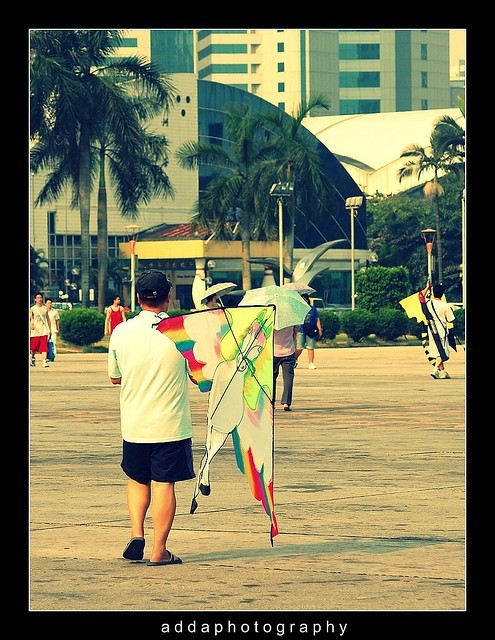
[125,223,142,312]
[345,196,363,311]
[421,228,437,280]
[269,181,293,287]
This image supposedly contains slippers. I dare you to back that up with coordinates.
[123,537,146,560]
[147,550,182,566]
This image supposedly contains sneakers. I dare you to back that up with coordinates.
[29,357,37,367]
[284,403,292,411]
[441,371,451,378]
[41,359,49,367]
[430,369,440,379]
[308,363,317,370]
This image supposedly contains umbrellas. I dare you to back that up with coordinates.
[279,283,317,296]
[199,282,237,307]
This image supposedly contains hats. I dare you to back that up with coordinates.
[136,269,173,299]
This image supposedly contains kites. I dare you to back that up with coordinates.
[150,304,279,548]
[399,287,465,367]
[238,285,312,331]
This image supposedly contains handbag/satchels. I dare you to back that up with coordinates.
[47,335,55,360]
[302,322,319,338]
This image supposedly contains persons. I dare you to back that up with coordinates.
[197,293,221,311]
[109,269,199,566]
[104,295,126,336]
[425,284,456,379]
[30,292,52,368]
[294,294,322,370]
[43,298,59,362]
[274,326,296,411]
